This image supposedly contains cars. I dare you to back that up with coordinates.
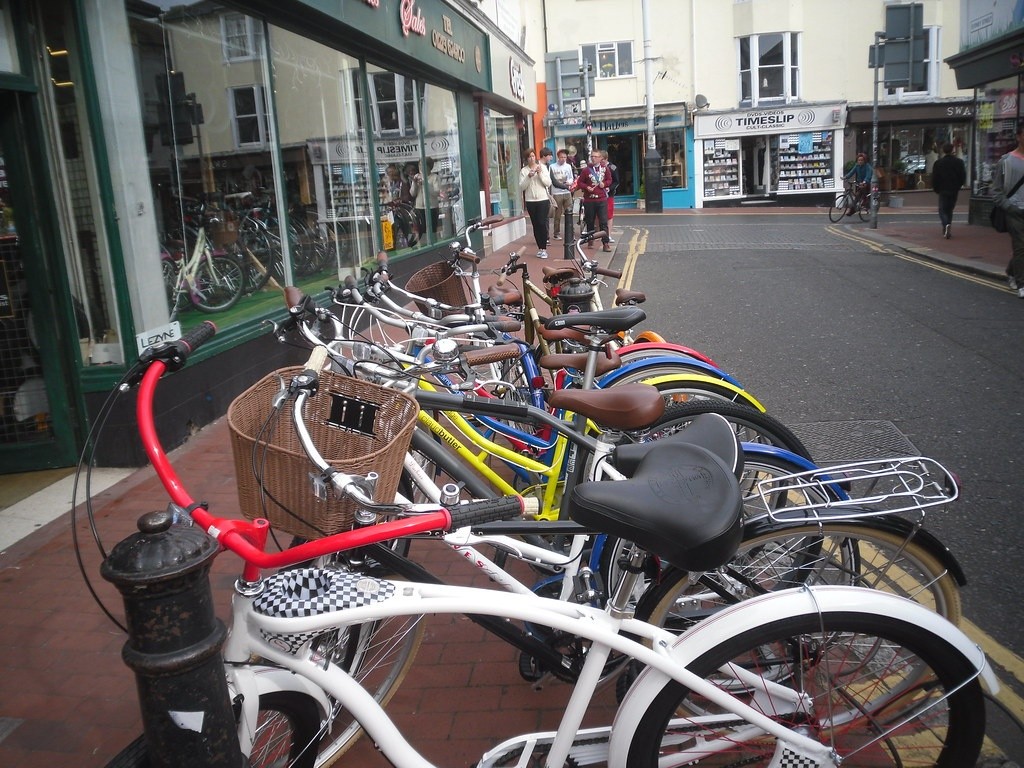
[901,154,927,175]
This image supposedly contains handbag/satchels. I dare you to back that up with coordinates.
[546,193,558,219]
[989,207,1008,233]
[595,188,614,220]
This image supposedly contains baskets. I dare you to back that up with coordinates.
[405,261,469,320]
[226,364,421,541]
[203,210,239,245]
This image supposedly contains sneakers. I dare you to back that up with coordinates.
[540,248,548,259]
[536,249,542,258]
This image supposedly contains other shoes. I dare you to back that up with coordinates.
[608,236,615,243]
[1007,275,1017,289]
[588,239,595,248]
[603,244,611,251]
[552,234,562,240]
[1018,288,1024,298]
[546,239,551,245]
[943,223,953,239]
[846,207,856,217]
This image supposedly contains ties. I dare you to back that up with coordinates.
[595,167,599,173]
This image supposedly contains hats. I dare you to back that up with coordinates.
[567,145,578,156]
[556,149,570,154]
[943,142,954,153]
[579,160,587,168]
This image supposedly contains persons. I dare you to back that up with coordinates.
[992,123,1024,298]
[933,144,967,238]
[577,149,619,252]
[386,156,440,250]
[518,148,576,258]
[842,153,872,216]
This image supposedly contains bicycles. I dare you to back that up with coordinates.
[160,190,426,316]
[829,177,881,223]
[68,216,1004,768]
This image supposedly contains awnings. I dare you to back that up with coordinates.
[848,106,976,125]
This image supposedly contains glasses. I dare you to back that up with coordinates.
[590,156,597,158]
